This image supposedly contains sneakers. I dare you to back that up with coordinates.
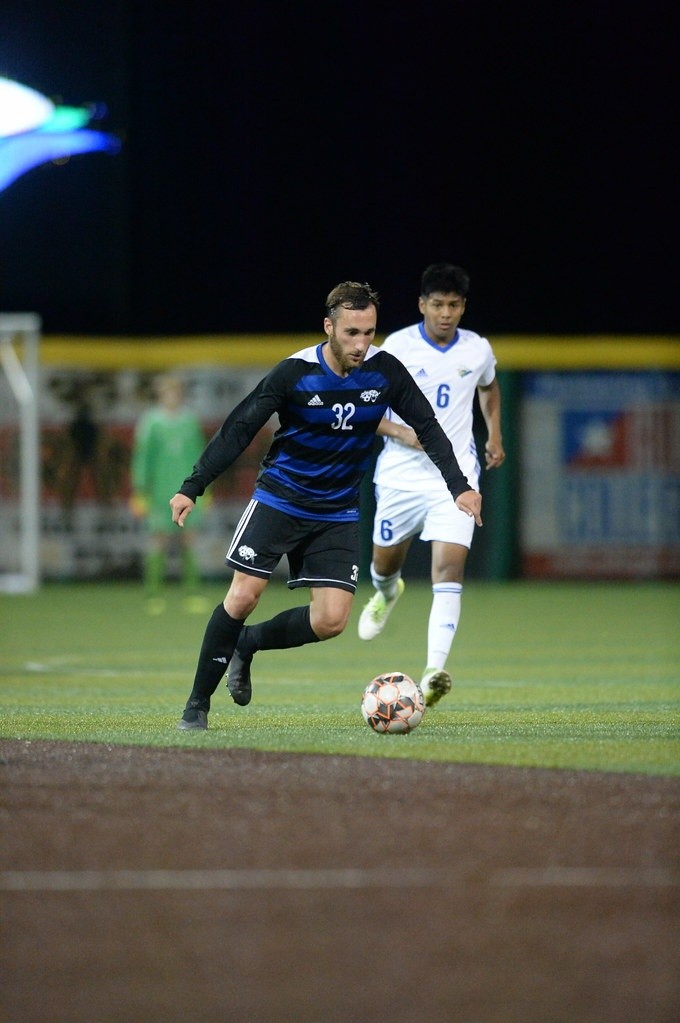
[176,694,210,731]
[419,670,451,709]
[225,625,252,705]
[358,578,404,640]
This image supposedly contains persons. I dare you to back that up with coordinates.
[130,370,208,614]
[358,265,506,708]
[169,283,482,733]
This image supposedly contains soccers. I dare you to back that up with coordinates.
[362,671,425,737]
